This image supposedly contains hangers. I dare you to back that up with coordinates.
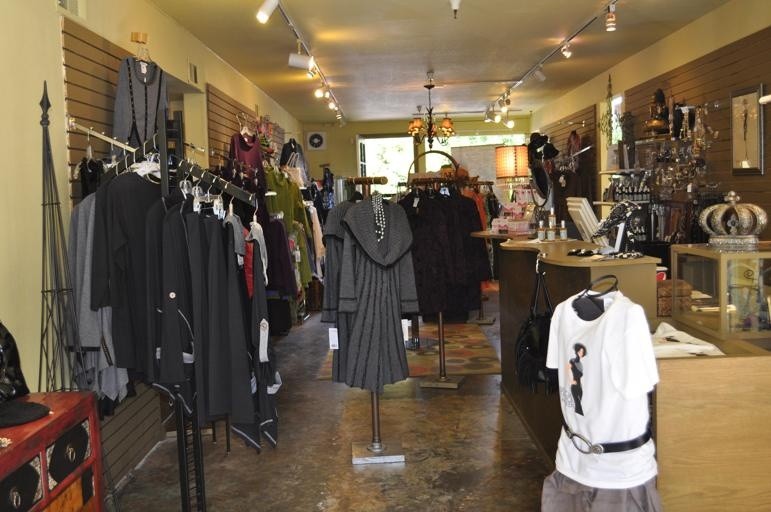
[85,111,451,241]
[133,42,152,64]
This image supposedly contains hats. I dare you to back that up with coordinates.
[527,133,548,150]
[543,143,558,158]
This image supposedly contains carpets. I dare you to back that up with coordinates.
[316,318,503,380]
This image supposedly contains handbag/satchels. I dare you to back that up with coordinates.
[528,151,548,207]
[515,272,559,396]
[280,166,304,187]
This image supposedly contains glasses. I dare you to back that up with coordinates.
[568,248,601,256]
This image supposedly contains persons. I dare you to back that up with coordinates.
[538,274,664,511]
[567,341,588,416]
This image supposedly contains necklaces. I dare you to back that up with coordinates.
[371,191,386,244]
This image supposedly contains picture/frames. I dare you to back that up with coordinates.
[729,82,765,177]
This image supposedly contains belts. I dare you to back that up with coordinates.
[561,416,651,453]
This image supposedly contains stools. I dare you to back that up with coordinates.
[658,279,692,316]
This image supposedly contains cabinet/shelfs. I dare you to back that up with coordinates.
[0,392,104,512]
[592,168,651,205]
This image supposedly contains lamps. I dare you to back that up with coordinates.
[495,145,528,179]
[483,0,618,128]
[407,72,456,151]
[257,1,343,119]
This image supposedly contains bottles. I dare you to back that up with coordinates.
[537,208,567,242]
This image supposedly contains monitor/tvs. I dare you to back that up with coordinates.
[566,196,600,243]
